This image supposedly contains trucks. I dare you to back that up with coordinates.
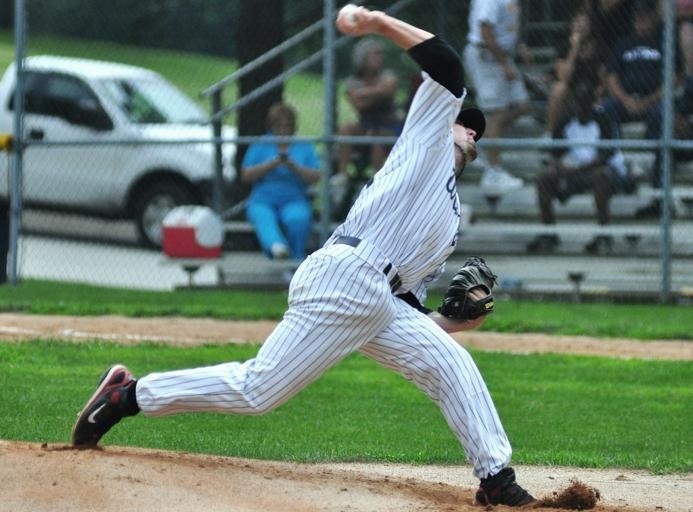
[0,53,238,250]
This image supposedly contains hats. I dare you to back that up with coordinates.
[455,108,487,143]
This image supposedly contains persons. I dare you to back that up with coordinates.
[73,4,538,507]
[328,35,399,188]
[242,101,322,283]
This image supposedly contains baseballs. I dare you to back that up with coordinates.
[338,4,359,26]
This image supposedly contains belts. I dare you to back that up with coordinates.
[332,235,403,294]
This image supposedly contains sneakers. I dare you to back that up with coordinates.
[71,363,138,446]
[474,466,538,507]
[526,234,561,253]
[585,235,612,257]
[468,156,525,196]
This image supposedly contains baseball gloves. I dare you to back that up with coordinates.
[436,256,495,320]
